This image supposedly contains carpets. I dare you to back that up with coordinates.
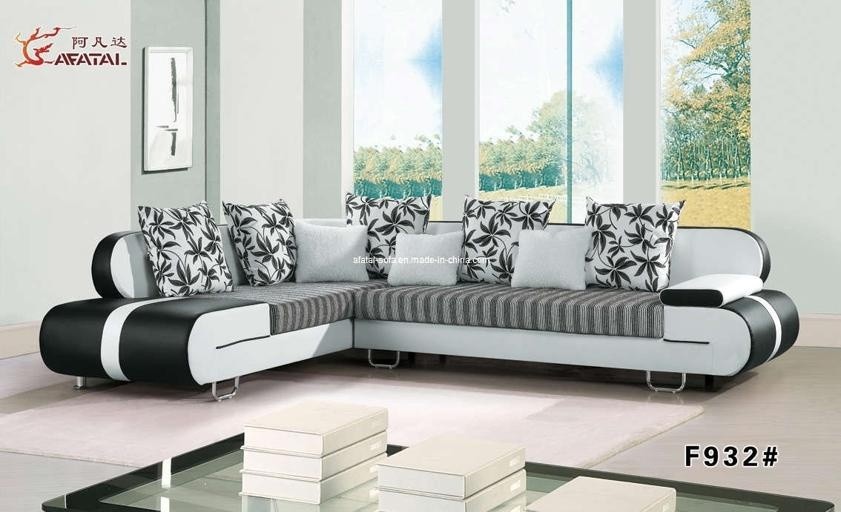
[0,369,703,469]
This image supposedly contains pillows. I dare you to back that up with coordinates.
[133,193,688,298]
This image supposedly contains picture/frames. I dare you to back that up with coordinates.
[141,47,196,174]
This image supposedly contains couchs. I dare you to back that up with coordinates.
[39,221,800,402]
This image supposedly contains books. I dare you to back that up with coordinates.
[237,403,677,512]
